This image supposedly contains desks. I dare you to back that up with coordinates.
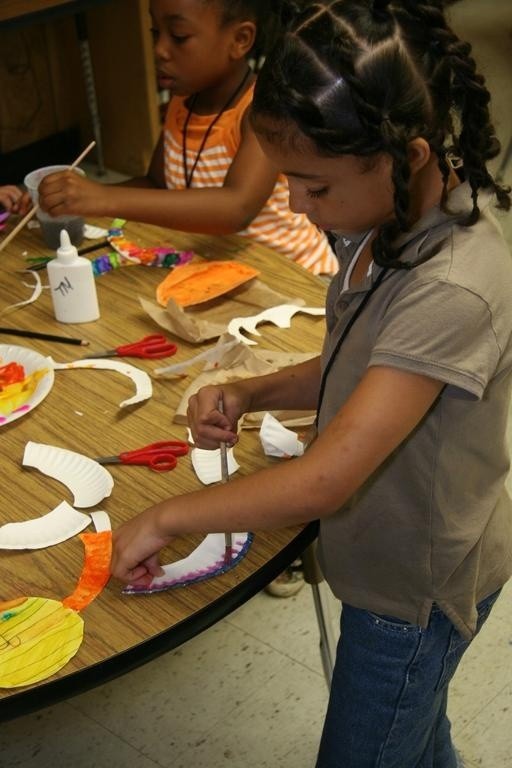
[0,199,334,725]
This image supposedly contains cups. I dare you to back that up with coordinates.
[25,165,89,248]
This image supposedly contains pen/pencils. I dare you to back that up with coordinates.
[25,241,110,273]
[0,327,89,346]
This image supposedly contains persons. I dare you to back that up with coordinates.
[36,0,342,600]
[103,0,512,767]
[1,181,23,212]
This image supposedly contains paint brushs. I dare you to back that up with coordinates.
[219,391,231,564]
[0,140,97,252]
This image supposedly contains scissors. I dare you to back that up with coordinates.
[83,333,177,360]
[93,440,189,470]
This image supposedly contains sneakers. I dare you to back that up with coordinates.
[264,558,305,598]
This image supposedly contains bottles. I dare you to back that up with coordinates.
[47,230,101,325]
